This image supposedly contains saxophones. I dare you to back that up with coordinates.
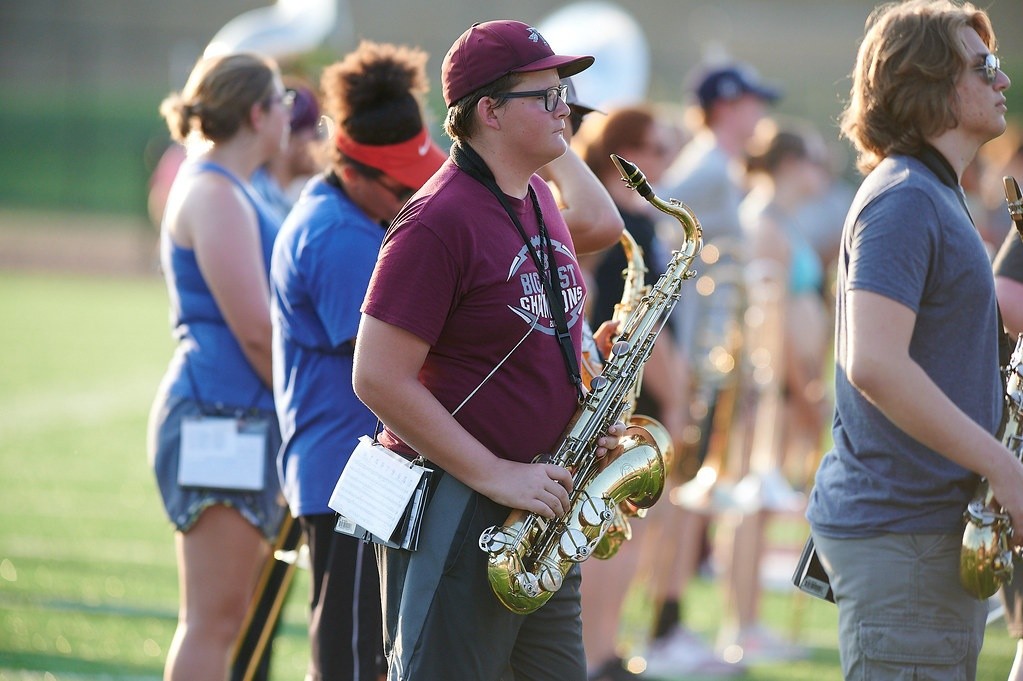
[471,148,707,617]
[956,173,1023,604]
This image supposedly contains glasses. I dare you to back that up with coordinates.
[471,84,568,111]
[948,54,1000,82]
[350,166,413,201]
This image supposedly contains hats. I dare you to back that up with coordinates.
[335,114,446,190]
[695,66,779,105]
[558,77,607,117]
[442,20,596,109]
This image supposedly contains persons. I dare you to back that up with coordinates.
[725,129,830,665]
[269,40,445,681]
[581,108,683,681]
[352,20,627,681]
[149,51,289,680]
[646,67,778,681]
[804,0,1023,681]
[244,87,327,222]
